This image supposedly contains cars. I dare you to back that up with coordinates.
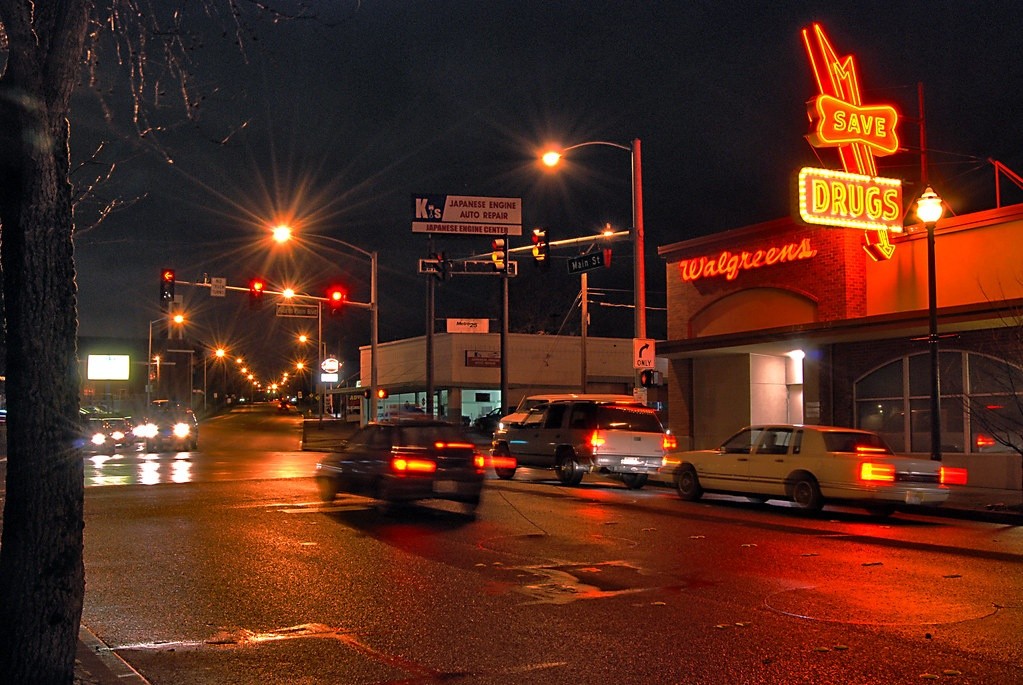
[314,422,490,515]
[662,422,974,521]
[79,403,145,458]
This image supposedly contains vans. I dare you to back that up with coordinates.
[488,394,634,455]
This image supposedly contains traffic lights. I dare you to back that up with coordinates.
[363,390,371,399]
[327,290,348,311]
[159,267,176,302]
[530,227,547,261]
[490,236,509,280]
[429,246,450,287]
[244,278,267,295]
[378,389,389,400]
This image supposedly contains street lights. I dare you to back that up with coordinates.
[582,224,616,394]
[269,224,379,428]
[282,290,328,419]
[540,138,647,390]
[914,182,943,464]
[298,335,330,415]
[147,313,190,411]
[203,348,225,411]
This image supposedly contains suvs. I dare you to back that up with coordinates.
[146,410,199,453]
[489,399,679,490]
